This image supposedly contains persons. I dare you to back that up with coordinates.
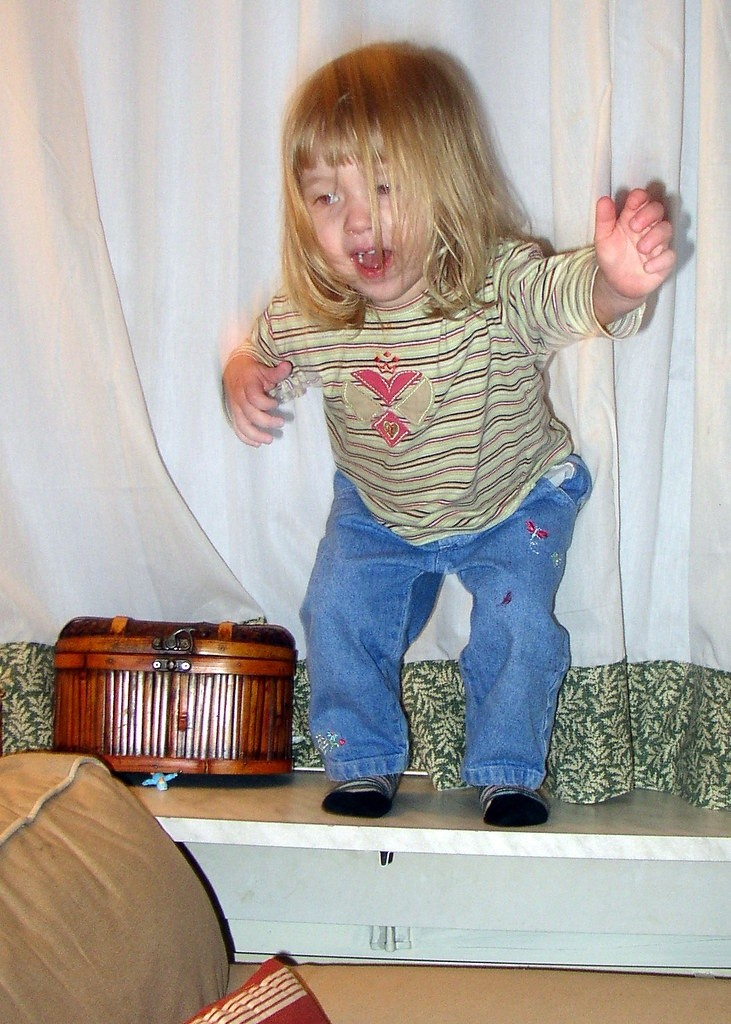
[220,40,676,828]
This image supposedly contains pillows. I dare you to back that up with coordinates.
[182,959,329,1024]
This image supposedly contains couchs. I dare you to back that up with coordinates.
[0,751,731,1024]
